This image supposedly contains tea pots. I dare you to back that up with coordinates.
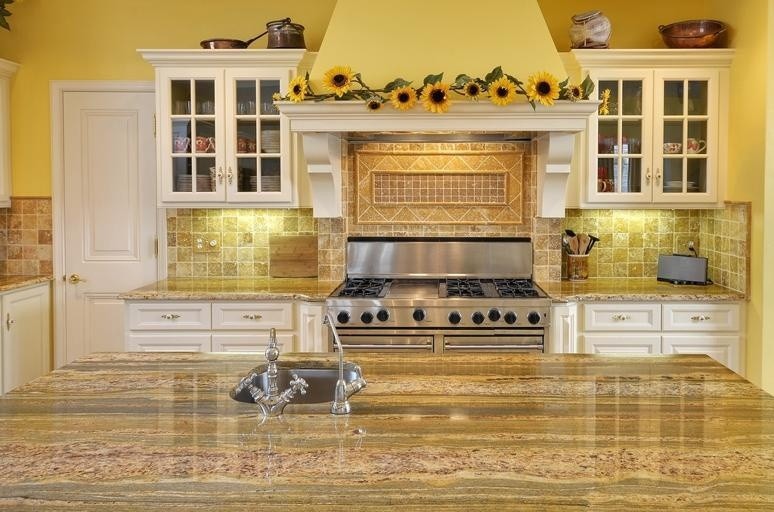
[687,138,706,154]
[266,17,306,48]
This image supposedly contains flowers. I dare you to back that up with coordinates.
[271,66,611,118]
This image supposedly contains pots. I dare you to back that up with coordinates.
[658,19,727,48]
[199,30,268,49]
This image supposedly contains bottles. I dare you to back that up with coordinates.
[569,8,611,49]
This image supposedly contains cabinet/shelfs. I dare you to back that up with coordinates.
[123,299,298,355]
[575,303,746,379]
[154,66,302,210]
[0,280,51,395]
[578,65,728,210]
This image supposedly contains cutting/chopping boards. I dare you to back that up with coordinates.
[268,235,318,277]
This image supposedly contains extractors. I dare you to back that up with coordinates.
[274,0,603,142]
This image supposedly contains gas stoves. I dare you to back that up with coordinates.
[325,277,553,329]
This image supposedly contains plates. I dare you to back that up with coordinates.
[261,130,280,153]
[664,180,699,192]
[177,166,281,192]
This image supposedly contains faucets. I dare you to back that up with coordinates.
[264,327,279,406]
[320,313,351,415]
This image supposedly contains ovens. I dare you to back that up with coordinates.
[327,330,545,353]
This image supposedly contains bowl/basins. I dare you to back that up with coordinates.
[664,142,682,154]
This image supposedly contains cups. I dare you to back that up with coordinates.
[567,254,589,281]
[174,100,278,115]
[598,179,614,192]
[172,136,256,154]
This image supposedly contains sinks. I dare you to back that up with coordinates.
[229,359,361,404]
